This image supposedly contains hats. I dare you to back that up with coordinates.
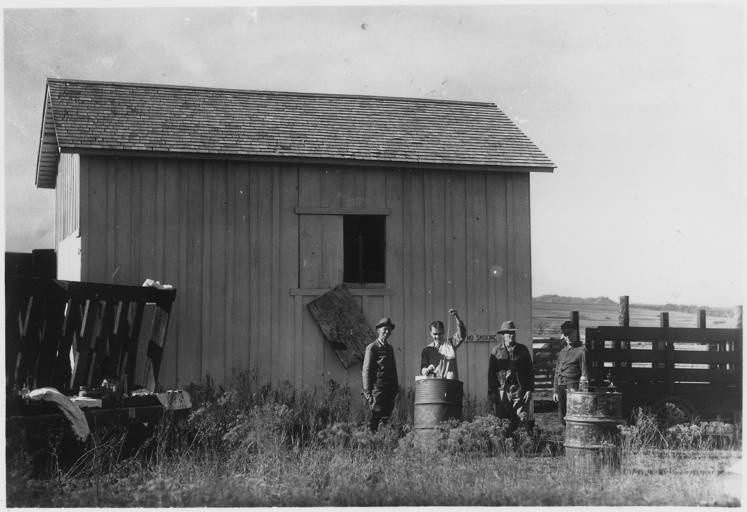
[496,321,519,334]
[375,317,395,330]
[561,321,578,336]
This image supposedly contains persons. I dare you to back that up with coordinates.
[485,319,535,433]
[550,320,592,430]
[418,307,467,382]
[360,316,400,436]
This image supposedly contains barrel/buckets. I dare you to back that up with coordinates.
[562,390,623,482]
[413,377,464,446]
